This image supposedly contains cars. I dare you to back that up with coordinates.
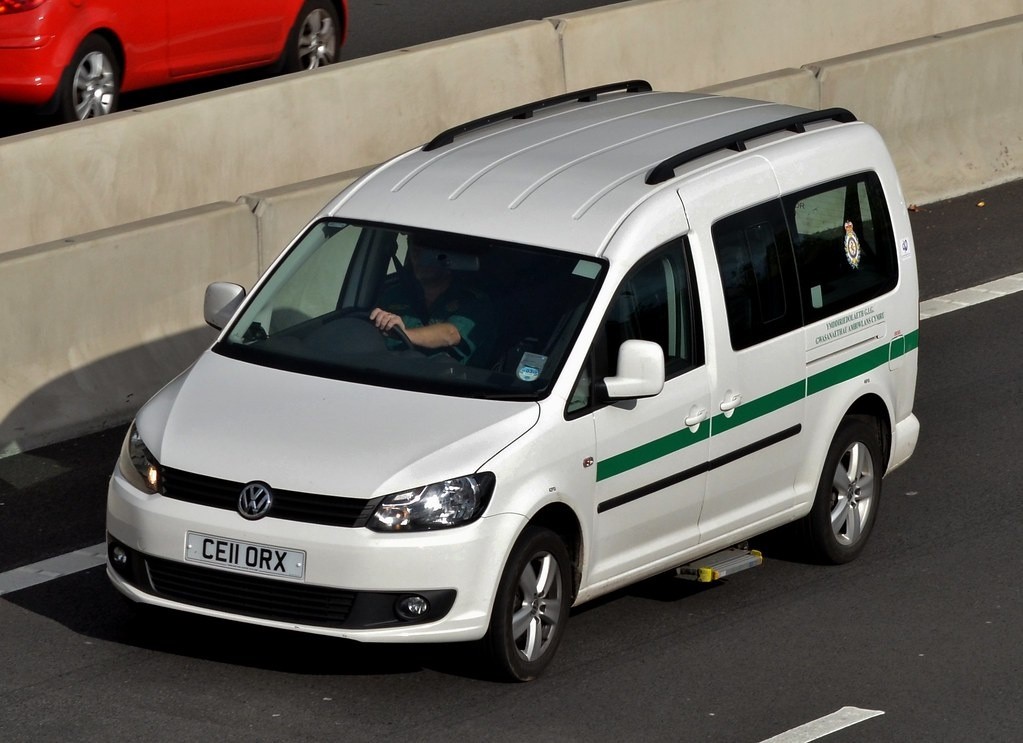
[1,0,349,126]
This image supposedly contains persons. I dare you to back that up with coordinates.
[369,235,496,369]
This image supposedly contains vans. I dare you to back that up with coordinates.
[105,81,925,677]
